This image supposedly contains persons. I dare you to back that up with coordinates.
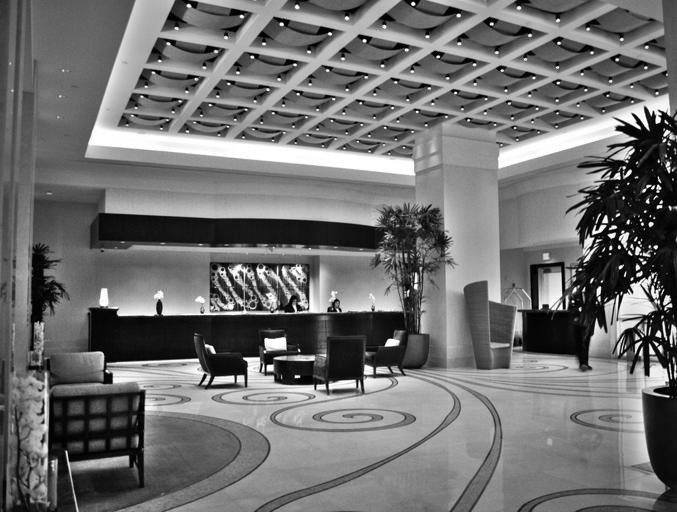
[285,295,302,312]
[325,299,342,312]
[566,283,596,370]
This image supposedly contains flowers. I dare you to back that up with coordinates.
[195,296,205,304]
[153,290,164,299]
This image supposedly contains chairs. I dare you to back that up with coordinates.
[464,280,516,371]
[49,348,145,495]
[194,329,408,396]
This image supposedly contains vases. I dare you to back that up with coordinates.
[200,305,205,314]
[156,300,162,316]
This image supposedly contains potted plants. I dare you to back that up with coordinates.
[370,205,457,369]
[551,108,676,491]
